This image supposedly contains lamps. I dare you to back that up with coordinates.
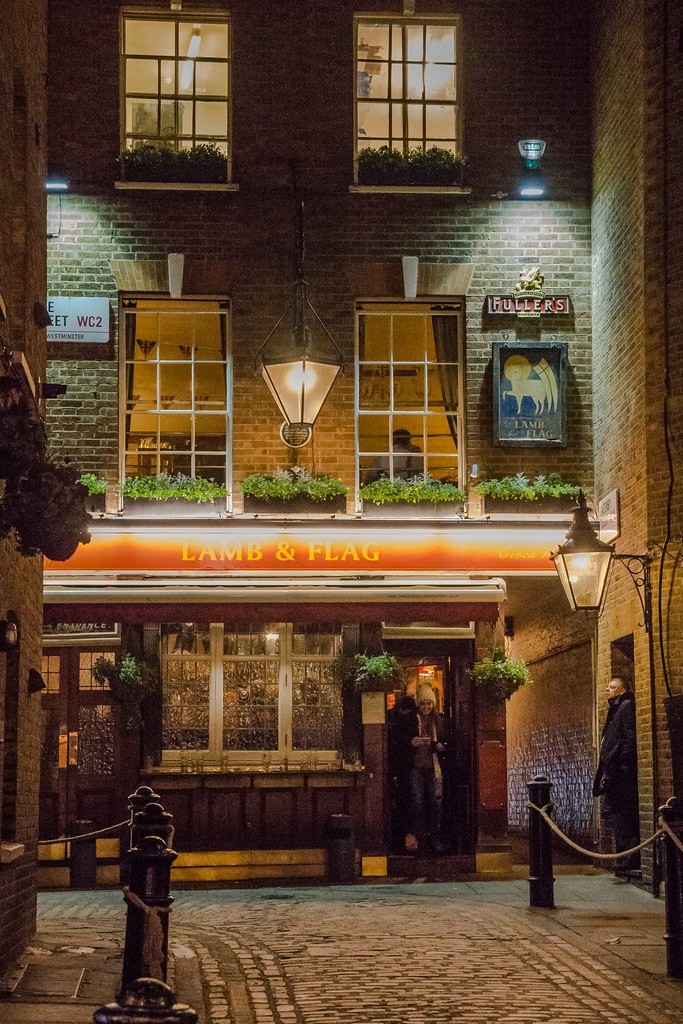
[254,165,346,452]
[0,619,17,651]
[519,138,547,197]
[179,27,202,89]
[547,490,651,633]
[471,462,480,478]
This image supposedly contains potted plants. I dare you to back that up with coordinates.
[477,478,590,512]
[90,653,157,708]
[469,645,529,699]
[76,473,106,513]
[243,466,346,515]
[117,141,229,184]
[119,476,228,515]
[351,652,406,691]
[360,475,466,518]
[358,146,473,186]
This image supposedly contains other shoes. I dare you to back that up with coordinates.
[433,838,449,852]
[613,853,641,871]
[405,833,419,851]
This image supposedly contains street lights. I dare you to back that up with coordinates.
[549,491,659,901]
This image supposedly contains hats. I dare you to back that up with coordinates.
[415,683,436,709]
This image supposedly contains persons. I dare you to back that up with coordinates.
[364,429,425,481]
[593,677,640,870]
[387,684,450,853]
